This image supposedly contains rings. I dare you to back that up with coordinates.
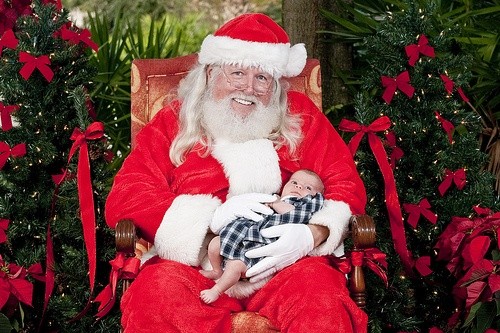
[274,266,277,272]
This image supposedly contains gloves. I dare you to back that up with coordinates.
[244,224,314,284]
[209,193,277,235]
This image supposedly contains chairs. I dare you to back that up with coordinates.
[113,52,377,333]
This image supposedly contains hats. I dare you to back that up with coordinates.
[198,14,307,78]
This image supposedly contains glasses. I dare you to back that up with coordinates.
[220,67,273,95]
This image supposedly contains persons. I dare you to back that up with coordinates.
[199,170,325,304]
[105,13,367,333]
